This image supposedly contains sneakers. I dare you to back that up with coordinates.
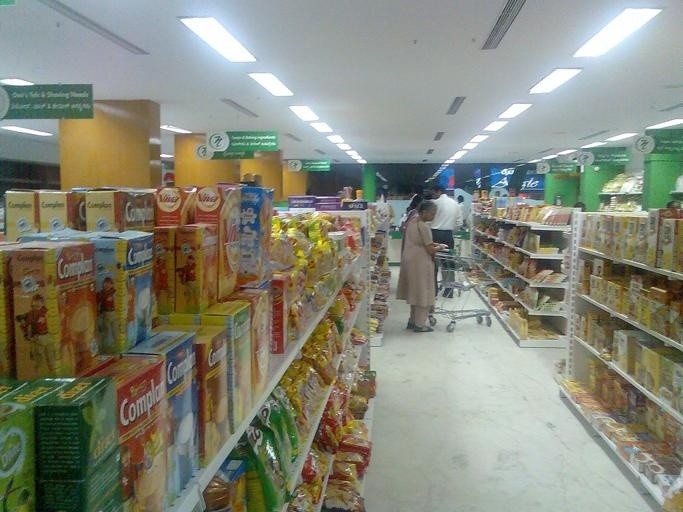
[406,322,433,334]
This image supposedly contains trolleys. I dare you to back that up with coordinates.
[426,245,499,332]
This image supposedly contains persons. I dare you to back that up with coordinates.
[425,183,463,299]
[400,185,422,218]
[393,200,448,333]
[455,194,470,232]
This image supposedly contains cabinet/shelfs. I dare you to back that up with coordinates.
[470,206,683,512]
[165,203,392,511]
